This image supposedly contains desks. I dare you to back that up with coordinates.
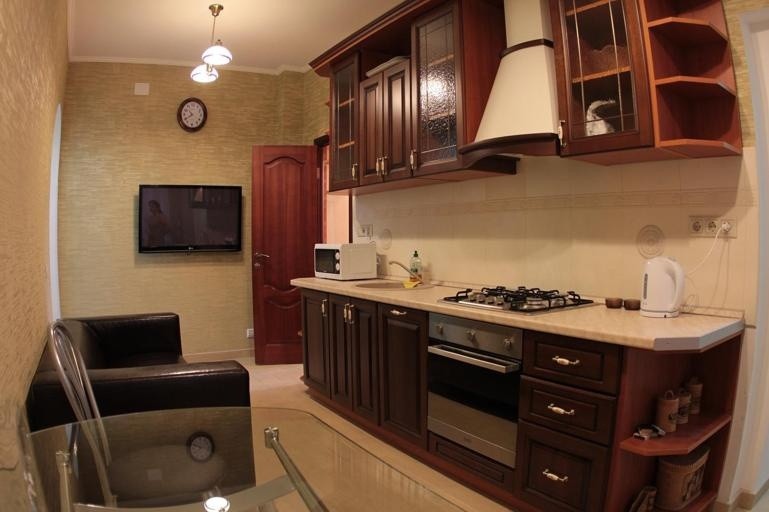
[0,404,468,512]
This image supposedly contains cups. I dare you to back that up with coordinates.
[655,382,704,434]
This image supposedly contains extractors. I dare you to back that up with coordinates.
[460,1,565,173]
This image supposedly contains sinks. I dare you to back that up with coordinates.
[355,279,442,291]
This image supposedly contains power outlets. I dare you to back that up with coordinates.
[688,214,738,240]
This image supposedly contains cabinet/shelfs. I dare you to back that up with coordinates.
[548,0,695,165]
[300,287,330,397]
[411,5,468,170]
[380,303,428,455]
[608,333,745,511]
[638,0,745,158]
[360,60,414,182]
[516,330,624,511]
[330,293,378,425]
[328,53,361,189]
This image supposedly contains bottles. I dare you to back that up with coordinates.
[408,250,422,284]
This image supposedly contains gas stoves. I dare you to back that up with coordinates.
[439,285,591,316]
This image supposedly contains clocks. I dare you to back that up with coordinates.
[176,98,208,133]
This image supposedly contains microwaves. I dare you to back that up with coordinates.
[314,241,377,281]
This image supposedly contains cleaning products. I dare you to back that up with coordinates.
[409,250,424,282]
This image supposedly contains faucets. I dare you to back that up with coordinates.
[387,260,428,285]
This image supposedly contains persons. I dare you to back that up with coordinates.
[146,200,168,249]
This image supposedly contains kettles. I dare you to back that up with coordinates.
[637,254,686,319]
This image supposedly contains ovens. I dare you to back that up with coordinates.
[425,337,522,452]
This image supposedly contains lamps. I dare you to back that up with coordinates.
[189,4,225,83]
[201,4,236,66]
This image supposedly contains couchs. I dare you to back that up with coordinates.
[26,310,257,511]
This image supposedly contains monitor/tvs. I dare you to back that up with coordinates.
[138,184,243,254]
